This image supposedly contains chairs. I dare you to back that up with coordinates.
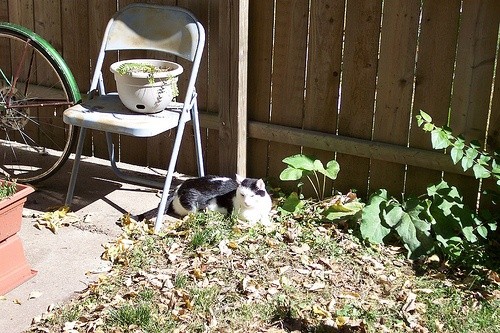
[63,4,205,236]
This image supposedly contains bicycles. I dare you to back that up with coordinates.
[0,21,82,185]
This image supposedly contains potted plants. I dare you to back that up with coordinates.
[0,177,35,243]
[110,60,183,114]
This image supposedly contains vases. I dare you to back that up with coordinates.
[0,235,38,297]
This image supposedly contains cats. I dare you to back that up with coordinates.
[167,174,273,226]
